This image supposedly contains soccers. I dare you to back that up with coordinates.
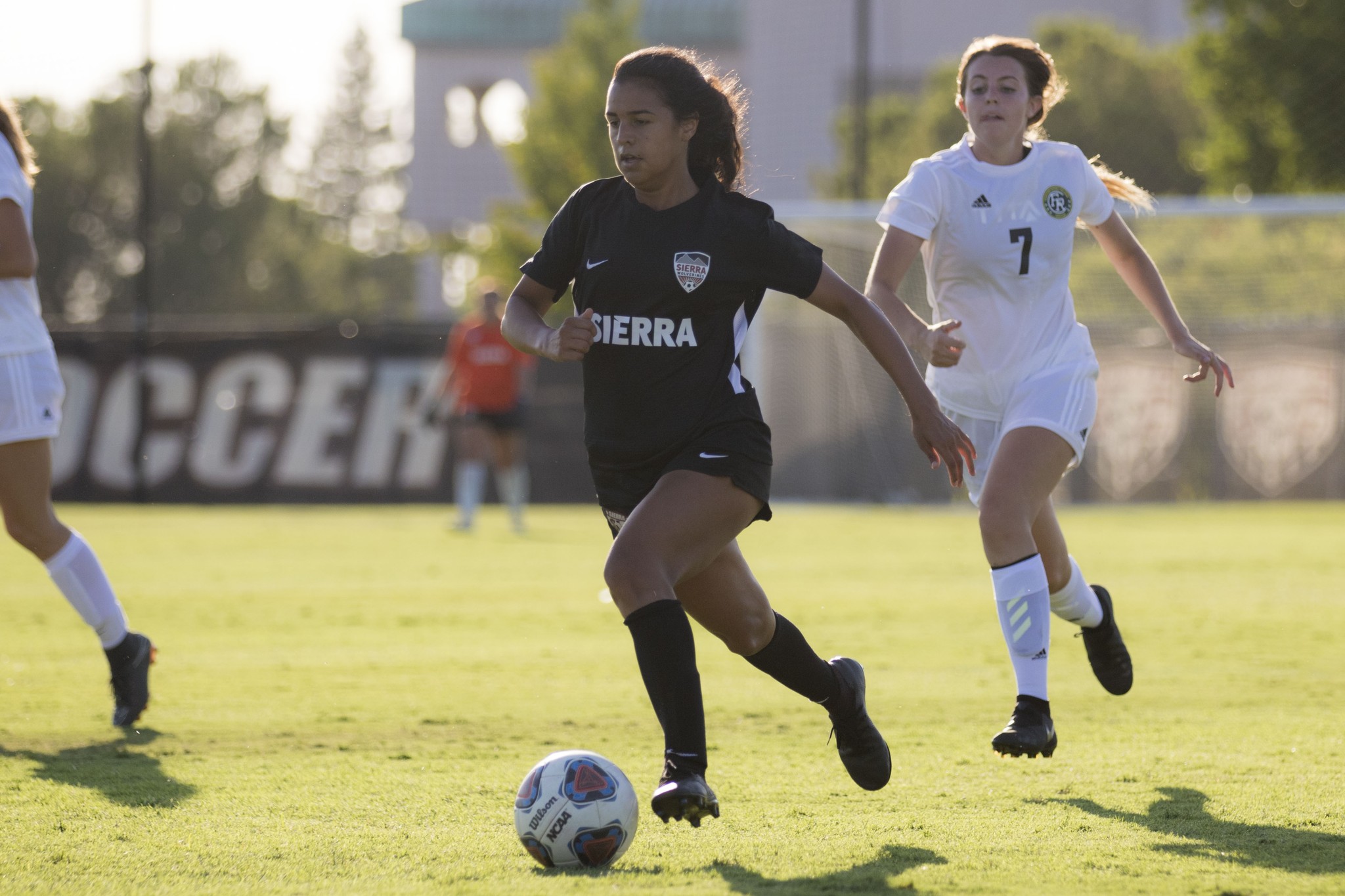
[514,752,640,872]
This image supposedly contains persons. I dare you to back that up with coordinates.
[1,105,158,729]
[862,33,1237,762]
[427,287,539,540]
[499,48,979,827]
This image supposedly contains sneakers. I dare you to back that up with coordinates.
[1074,585,1134,696]
[651,759,721,828]
[991,699,1057,759]
[108,632,158,727]
[823,656,892,791]
